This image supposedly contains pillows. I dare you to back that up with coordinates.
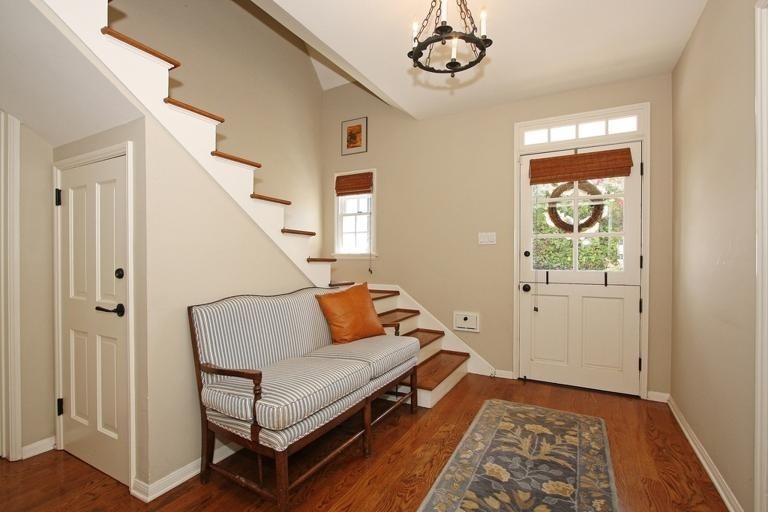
[315,282,386,345]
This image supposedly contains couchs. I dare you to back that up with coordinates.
[188,282,417,512]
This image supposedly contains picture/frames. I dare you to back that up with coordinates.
[341,116,368,156]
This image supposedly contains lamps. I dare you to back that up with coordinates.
[409,1,492,77]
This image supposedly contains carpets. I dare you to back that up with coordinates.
[416,399,621,512]
